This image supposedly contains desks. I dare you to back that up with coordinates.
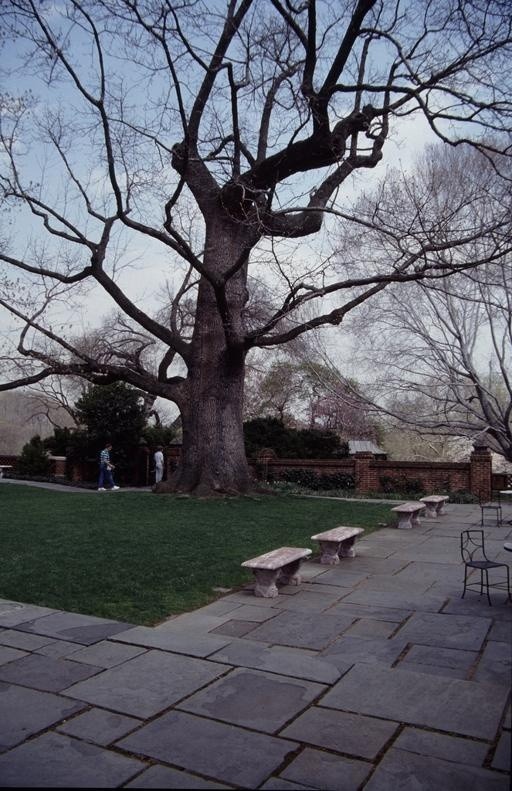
[504,543,512,604]
[499,490,512,525]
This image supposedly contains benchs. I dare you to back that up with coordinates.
[390,503,430,529]
[311,526,364,564]
[419,495,450,518]
[241,547,312,598]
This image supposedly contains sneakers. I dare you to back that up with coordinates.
[111,486,120,491]
[98,488,107,491]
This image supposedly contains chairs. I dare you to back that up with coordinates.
[479,489,502,528]
[461,530,512,606]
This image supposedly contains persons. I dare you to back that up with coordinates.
[153,446,165,483]
[98,444,120,491]
